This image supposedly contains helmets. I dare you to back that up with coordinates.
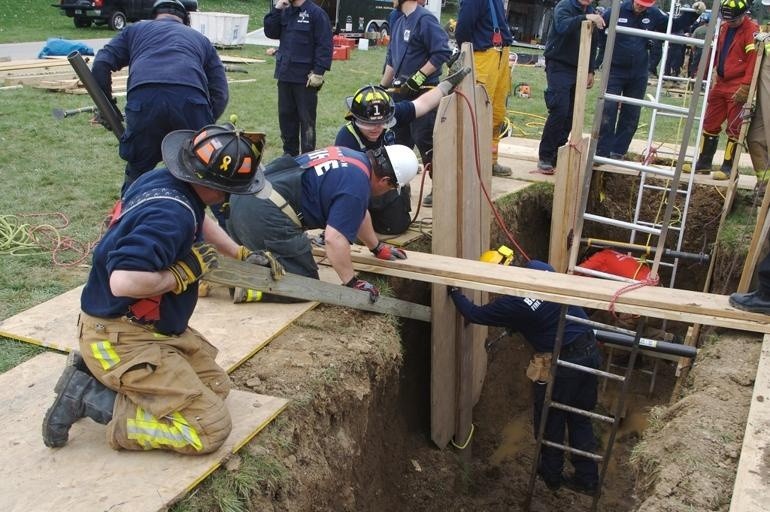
[635,0,656,7]
[151,0,186,10]
[479,245,514,266]
[384,144,419,196]
[160,121,265,194]
[345,82,395,124]
[718,0,750,21]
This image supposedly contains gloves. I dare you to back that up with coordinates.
[400,69,427,99]
[586,14,606,30]
[438,50,471,96]
[731,83,749,105]
[752,32,770,44]
[370,241,407,261]
[237,245,286,280]
[306,74,323,88]
[168,240,218,295]
[346,276,380,303]
[97,104,124,131]
[692,2,706,14]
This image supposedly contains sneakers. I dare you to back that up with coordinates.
[537,459,560,490]
[564,471,601,495]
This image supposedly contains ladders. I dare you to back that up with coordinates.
[523,0,721,510]
[601,0,720,396]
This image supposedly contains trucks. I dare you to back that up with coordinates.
[312,0,395,46]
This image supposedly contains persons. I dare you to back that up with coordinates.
[334,51,471,234]
[747,36,770,207]
[690,13,710,77]
[41,124,265,454]
[199,145,418,305]
[537,1,606,173]
[389,1,425,28]
[264,1,332,154]
[596,0,706,158]
[91,0,229,202]
[682,0,760,180]
[728,252,769,313]
[449,245,601,492]
[380,0,451,207]
[455,1,514,175]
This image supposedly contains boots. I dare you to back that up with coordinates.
[712,137,737,179]
[42,365,117,449]
[66,350,94,377]
[682,131,720,175]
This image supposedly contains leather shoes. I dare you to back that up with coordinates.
[493,164,511,177]
[422,188,433,207]
[537,160,553,174]
[729,289,770,315]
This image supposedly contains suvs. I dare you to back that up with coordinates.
[59,0,197,30]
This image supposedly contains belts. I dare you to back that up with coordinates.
[269,190,302,227]
